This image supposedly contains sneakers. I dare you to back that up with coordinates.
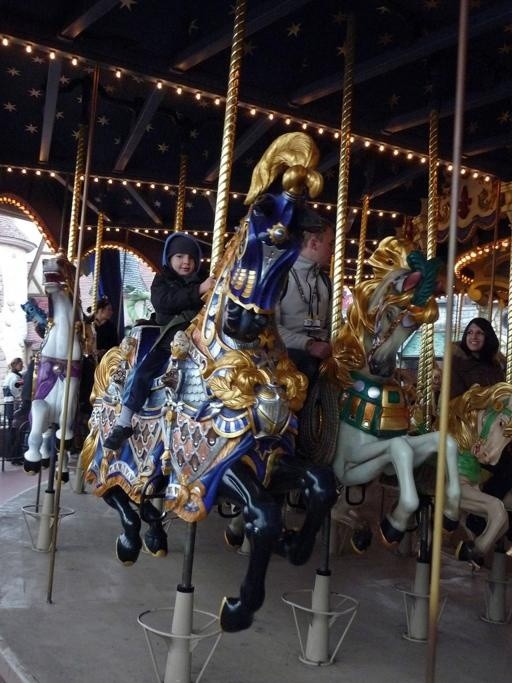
[102,424,135,451]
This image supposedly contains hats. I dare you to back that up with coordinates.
[167,237,200,269]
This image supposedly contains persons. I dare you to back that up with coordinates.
[438,311,509,390]
[89,298,121,358]
[268,214,343,397]
[3,356,34,467]
[100,226,219,451]
